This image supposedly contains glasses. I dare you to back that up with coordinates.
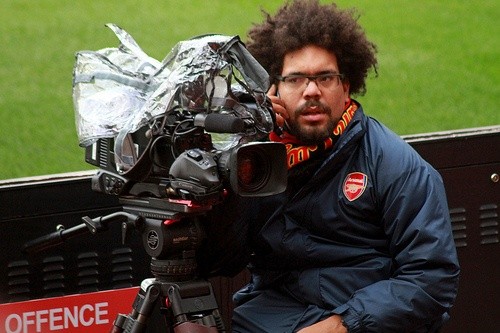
[275,73,344,87]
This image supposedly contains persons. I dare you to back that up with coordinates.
[197,0,459,333]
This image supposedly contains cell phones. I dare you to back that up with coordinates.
[275,91,294,134]
